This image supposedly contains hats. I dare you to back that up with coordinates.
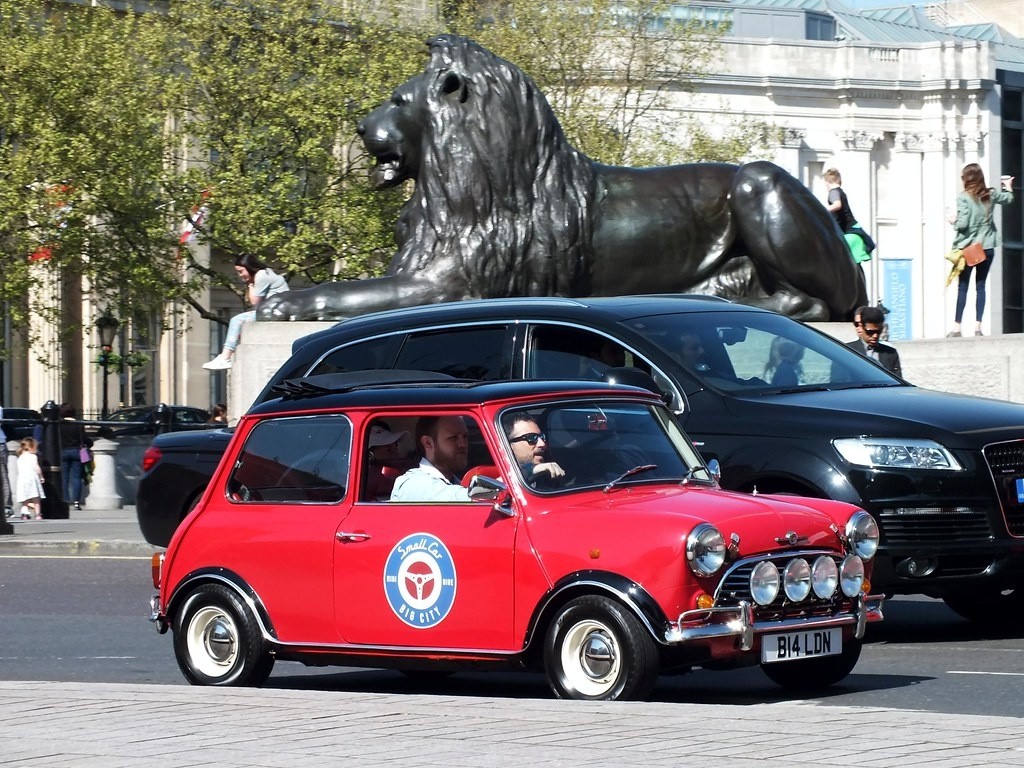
[5,440,21,451]
[367,425,410,448]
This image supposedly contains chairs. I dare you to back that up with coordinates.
[364,456,402,501]
[458,447,502,488]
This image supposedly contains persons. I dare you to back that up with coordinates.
[0,402,93,520]
[652,327,736,394]
[944,162,1015,339]
[365,413,567,504]
[579,335,629,379]
[766,167,904,390]
[207,405,227,429]
[202,254,290,370]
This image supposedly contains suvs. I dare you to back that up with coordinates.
[137,293,1024,638]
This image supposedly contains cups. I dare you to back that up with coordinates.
[1001,175,1011,189]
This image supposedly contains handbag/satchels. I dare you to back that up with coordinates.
[80,448,91,463]
[962,242,987,266]
[841,234,870,264]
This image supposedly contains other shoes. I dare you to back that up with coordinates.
[946,331,961,337]
[21,516,28,520]
[35,516,42,520]
[75,503,81,510]
[5,510,15,518]
[976,331,983,336]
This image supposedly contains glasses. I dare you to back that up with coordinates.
[864,327,883,335]
[854,321,862,327]
[508,433,546,446]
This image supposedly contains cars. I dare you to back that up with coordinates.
[1,408,42,440]
[105,404,211,435]
[150,372,885,702]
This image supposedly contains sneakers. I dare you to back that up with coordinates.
[202,354,232,370]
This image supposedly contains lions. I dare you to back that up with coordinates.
[255,34,870,322]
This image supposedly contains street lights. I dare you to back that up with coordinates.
[95,302,120,436]
